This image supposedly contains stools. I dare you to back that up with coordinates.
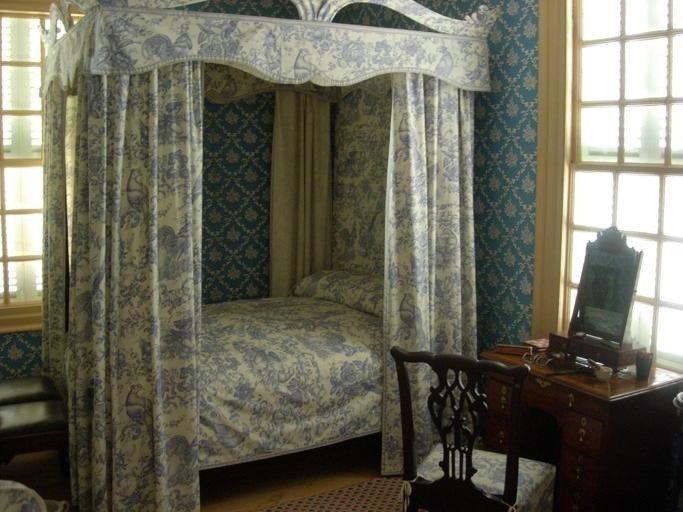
[0,399,68,476]
[0,375,61,405]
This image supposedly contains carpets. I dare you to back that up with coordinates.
[254,472,404,512]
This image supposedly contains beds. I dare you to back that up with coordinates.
[37,1,505,512]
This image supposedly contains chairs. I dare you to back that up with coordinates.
[390,345,557,511]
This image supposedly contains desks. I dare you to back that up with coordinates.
[479,347,683,511]
